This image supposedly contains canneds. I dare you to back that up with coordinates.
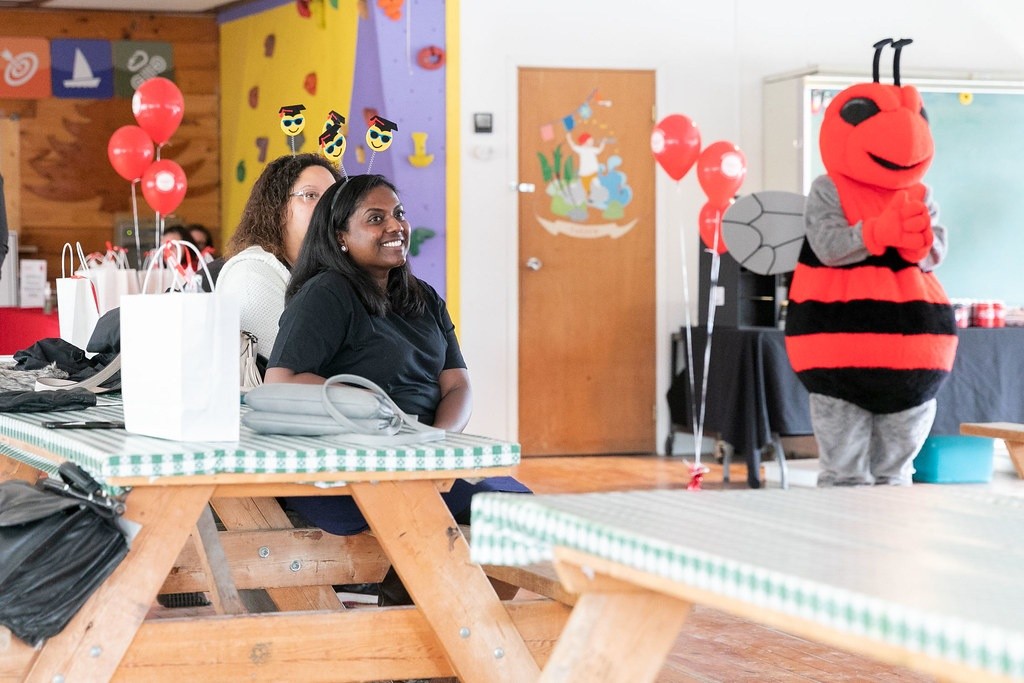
[953,302,1003,328]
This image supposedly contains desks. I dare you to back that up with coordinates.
[4,316,1022,683]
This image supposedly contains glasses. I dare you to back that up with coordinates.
[288,188,322,204]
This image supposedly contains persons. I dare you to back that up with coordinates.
[160,225,208,272]
[183,223,218,264]
[214,152,344,387]
[262,174,532,535]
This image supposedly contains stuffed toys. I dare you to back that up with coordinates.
[783,37,957,486]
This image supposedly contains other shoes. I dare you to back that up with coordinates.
[333,583,379,604]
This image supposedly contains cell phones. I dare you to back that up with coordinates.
[43,420,125,430]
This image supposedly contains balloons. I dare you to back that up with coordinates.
[140,158,187,216]
[131,76,184,146]
[699,198,735,254]
[651,113,700,180]
[107,124,154,183]
[696,140,746,210]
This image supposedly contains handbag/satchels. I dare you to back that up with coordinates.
[244,374,444,444]
[53,237,240,444]
[1,462,126,648]
